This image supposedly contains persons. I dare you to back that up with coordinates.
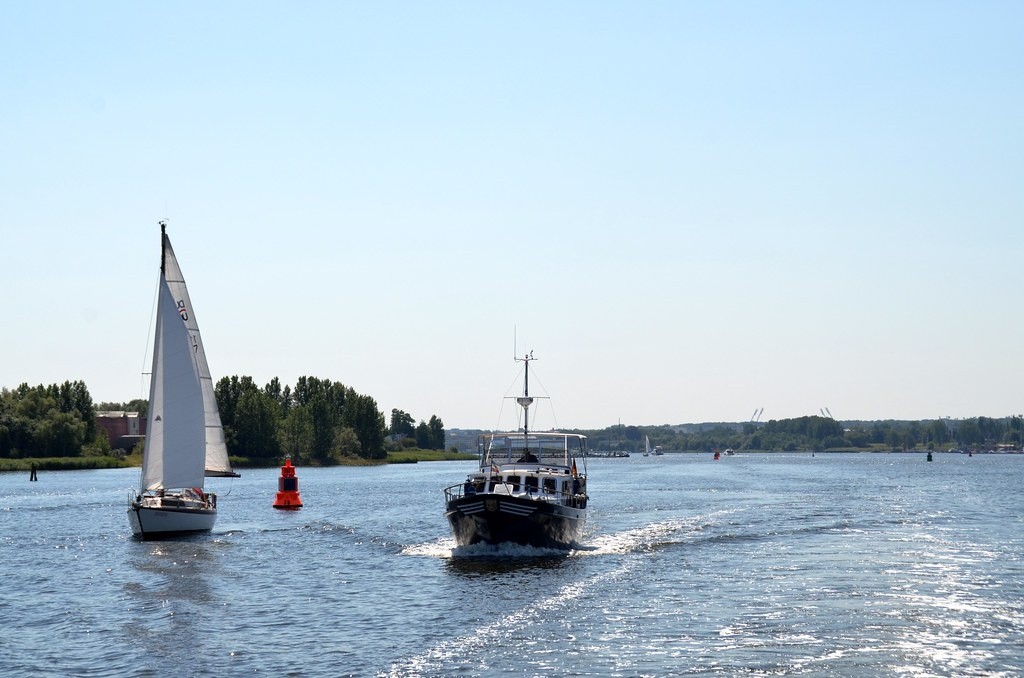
[475,482,483,492]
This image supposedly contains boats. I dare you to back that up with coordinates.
[724,449,734,455]
[651,446,664,455]
[442,324,590,551]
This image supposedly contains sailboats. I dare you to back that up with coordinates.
[643,435,652,456]
[127,220,240,534]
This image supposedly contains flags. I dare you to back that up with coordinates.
[491,460,501,473]
[572,459,577,480]
